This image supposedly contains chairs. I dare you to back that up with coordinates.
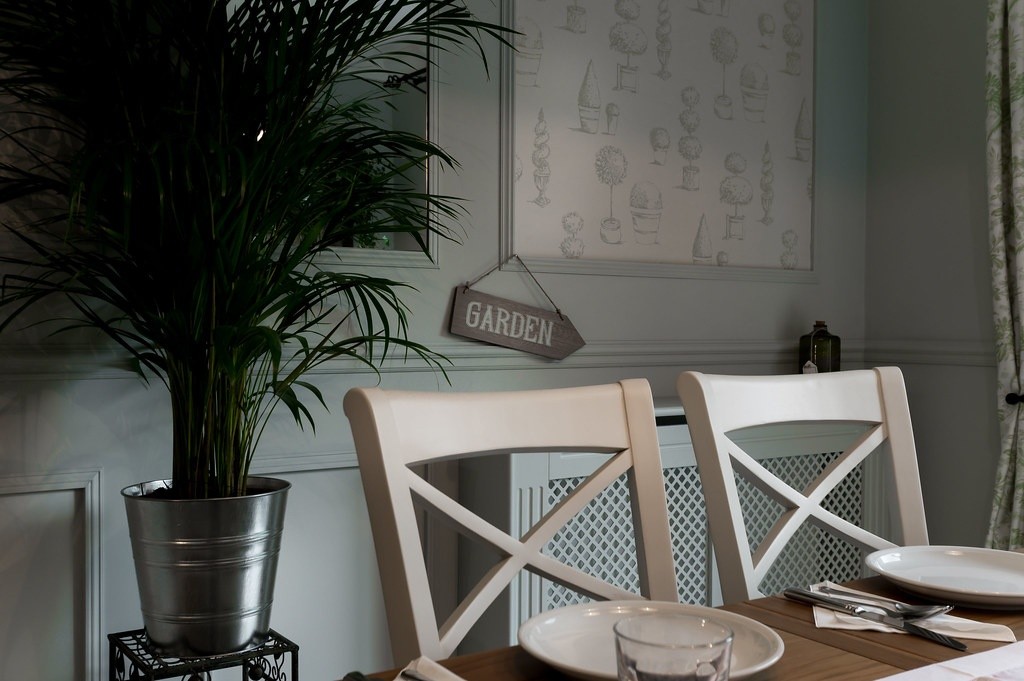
[677,365,929,607]
[343,378,680,670]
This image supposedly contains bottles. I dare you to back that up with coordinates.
[800,321,841,374]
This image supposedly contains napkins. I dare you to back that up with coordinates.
[391,656,466,681]
[809,578,1019,642]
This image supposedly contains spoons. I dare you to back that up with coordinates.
[818,587,950,611]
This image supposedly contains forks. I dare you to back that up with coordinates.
[841,606,954,622]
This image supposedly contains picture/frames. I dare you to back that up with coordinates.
[498,1,828,286]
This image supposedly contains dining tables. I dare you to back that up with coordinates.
[349,575,1024,681]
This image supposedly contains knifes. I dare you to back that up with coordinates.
[784,586,968,651]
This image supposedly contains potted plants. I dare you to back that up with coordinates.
[0,0,521,655]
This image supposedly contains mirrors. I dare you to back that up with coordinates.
[225,0,441,268]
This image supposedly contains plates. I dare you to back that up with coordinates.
[865,545,1024,610]
[518,601,784,679]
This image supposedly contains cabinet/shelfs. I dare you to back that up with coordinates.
[457,406,892,658]
[107,628,299,681]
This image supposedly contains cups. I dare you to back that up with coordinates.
[613,615,734,681]
